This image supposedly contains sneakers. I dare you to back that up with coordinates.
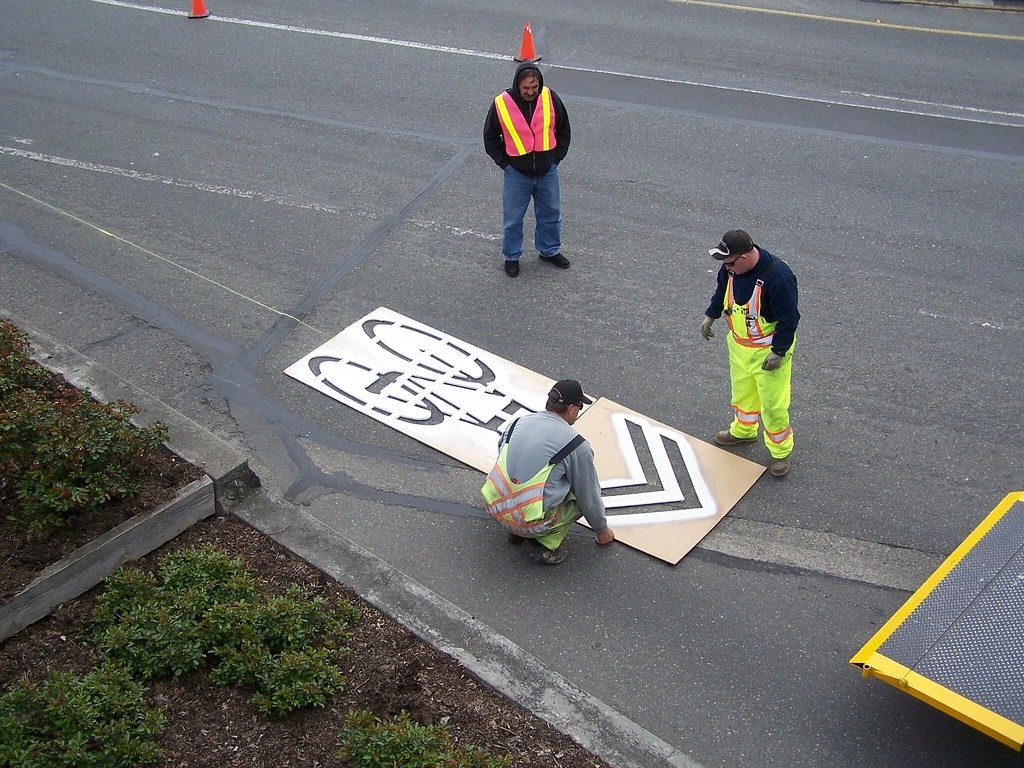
[714,427,758,446]
[771,456,790,476]
[521,539,568,563]
[505,260,519,277]
[508,531,525,544]
[538,252,570,268]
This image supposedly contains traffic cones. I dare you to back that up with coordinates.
[188,0,210,19]
[512,21,542,63]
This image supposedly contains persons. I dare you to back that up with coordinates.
[483,62,572,276]
[700,231,801,477]
[481,381,615,565]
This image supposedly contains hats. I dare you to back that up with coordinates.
[707,230,754,260]
[548,379,592,405]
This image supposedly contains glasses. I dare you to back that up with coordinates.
[723,254,742,267]
[574,402,583,409]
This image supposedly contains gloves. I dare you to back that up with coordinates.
[700,316,715,341]
[761,353,782,370]
